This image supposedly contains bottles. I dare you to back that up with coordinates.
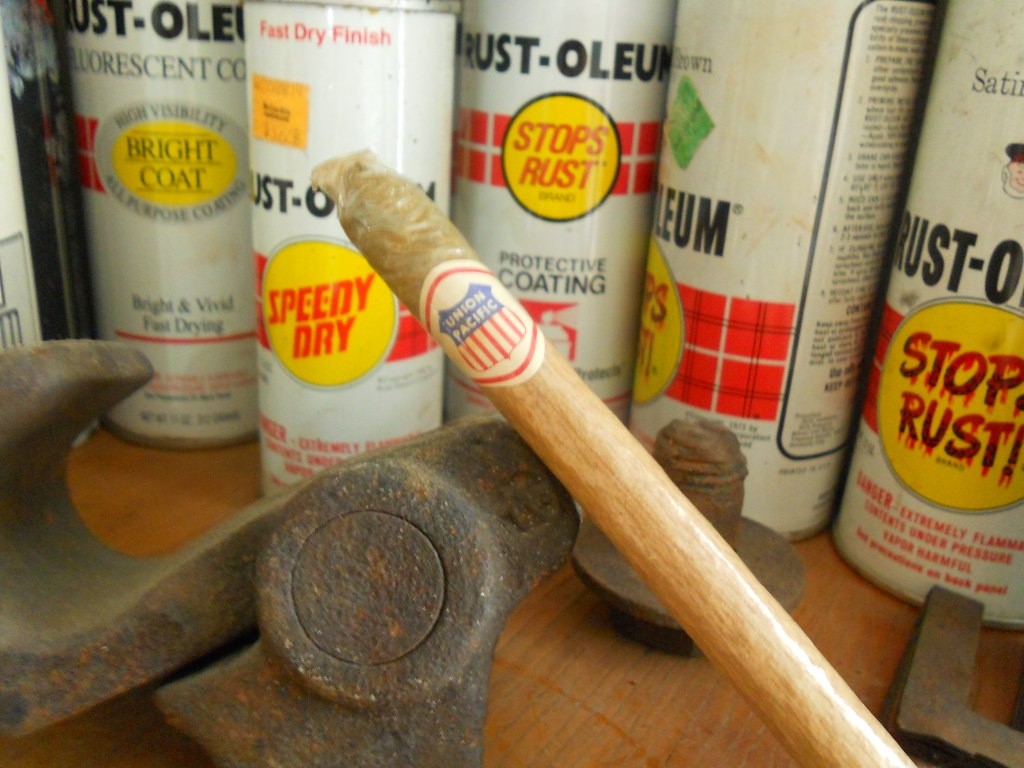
[242,1,463,496]
[0,0,98,348]
[66,0,259,450]
[447,0,683,438]
[831,0,1024,633]
[628,0,941,548]
